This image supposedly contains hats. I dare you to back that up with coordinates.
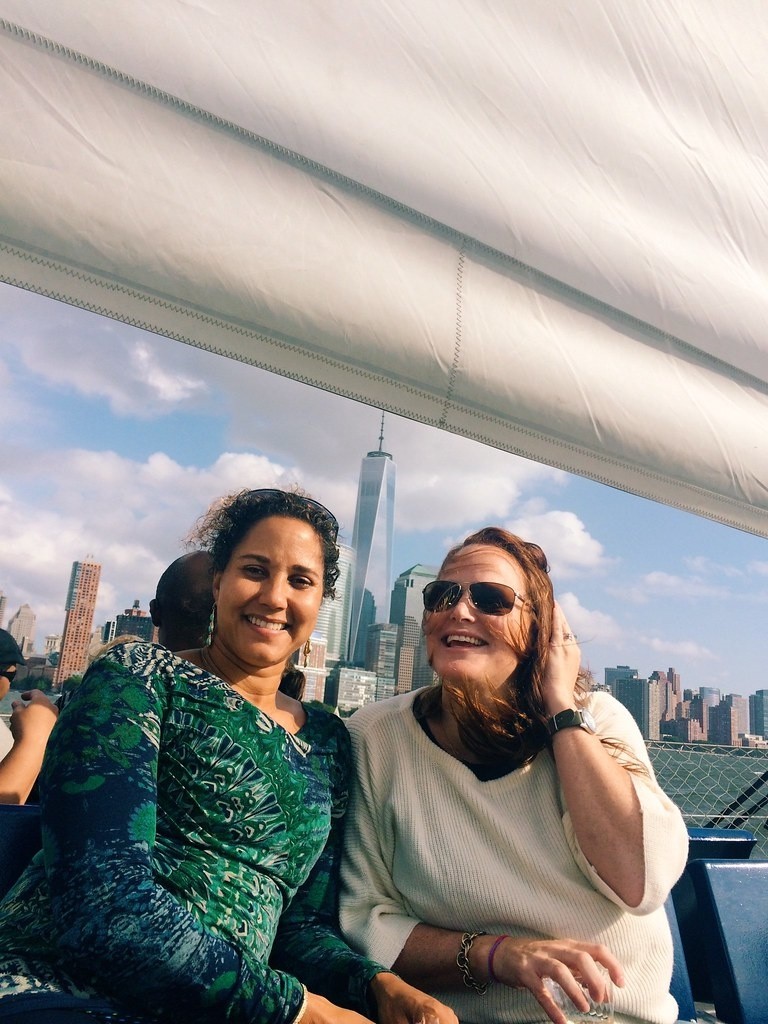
[0,628,27,666]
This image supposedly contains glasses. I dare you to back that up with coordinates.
[422,580,536,616]
[248,489,339,543]
[0,670,17,682]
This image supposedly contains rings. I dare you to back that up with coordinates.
[563,633,573,643]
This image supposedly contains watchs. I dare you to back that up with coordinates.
[545,709,596,734]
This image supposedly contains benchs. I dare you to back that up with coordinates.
[0,804,759,1024]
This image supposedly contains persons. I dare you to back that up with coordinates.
[340,526,690,1024]
[0,487,461,1024]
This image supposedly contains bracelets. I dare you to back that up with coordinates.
[488,934,512,985]
[457,929,492,995]
